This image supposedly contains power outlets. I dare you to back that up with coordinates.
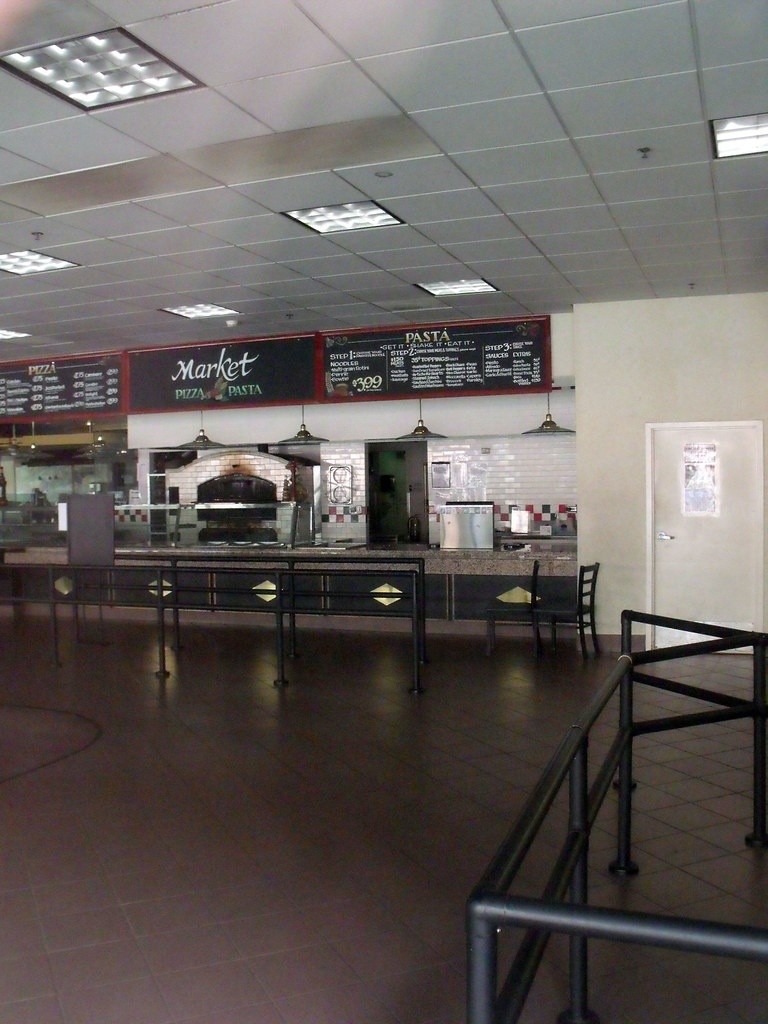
[481,448,490,454]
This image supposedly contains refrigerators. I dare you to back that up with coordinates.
[439,503,494,551]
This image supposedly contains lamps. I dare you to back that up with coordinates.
[0,418,128,460]
[520,393,577,434]
[278,405,330,444]
[395,398,448,440]
[177,409,227,450]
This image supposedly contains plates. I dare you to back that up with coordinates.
[209,542,227,545]
[234,542,251,545]
[260,542,278,544]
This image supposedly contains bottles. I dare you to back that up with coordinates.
[566,514,574,533]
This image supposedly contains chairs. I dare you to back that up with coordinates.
[483,559,543,659]
[534,562,601,659]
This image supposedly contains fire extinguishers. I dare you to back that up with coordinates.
[407,513,422,544]
